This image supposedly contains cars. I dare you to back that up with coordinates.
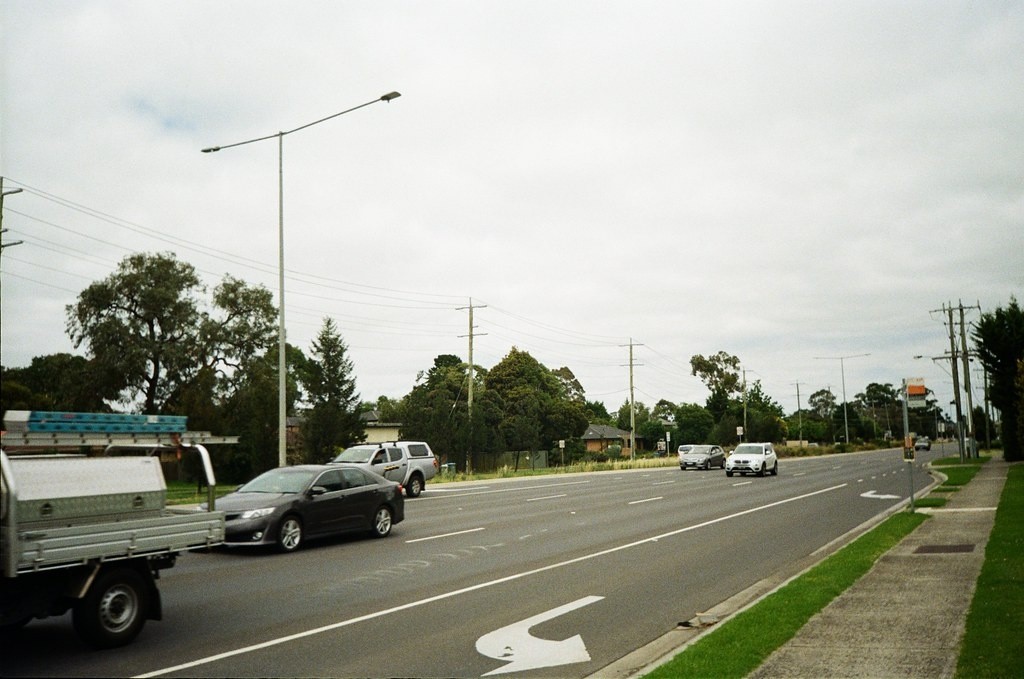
[808,443,819,447]
[915,438,931,451]
[726,443,778,476]
[200,464,404,555]
[679,445,726,470]
[678,445,696,455]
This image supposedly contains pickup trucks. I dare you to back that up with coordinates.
[325,441,438,499]
[0,444,227,652]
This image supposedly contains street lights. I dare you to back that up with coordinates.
[200,91,401,469]
[811,352,871,444]
